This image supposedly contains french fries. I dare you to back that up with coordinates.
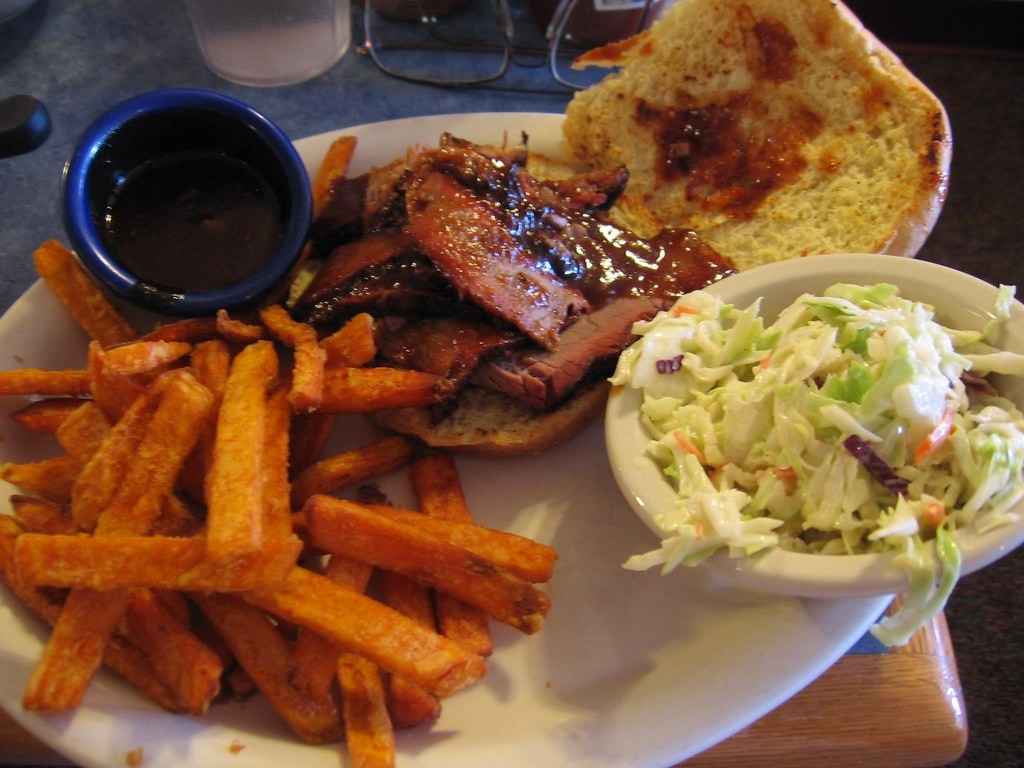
[0,132,561,768]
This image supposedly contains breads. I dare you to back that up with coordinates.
[368,367,612,462]
[561,0,954,265]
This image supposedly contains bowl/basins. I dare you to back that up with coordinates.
[604,254,1024,595]
[60,89,313,314]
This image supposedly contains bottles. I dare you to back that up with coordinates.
[185,0,351,88]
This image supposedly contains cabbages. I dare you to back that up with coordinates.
[608,284,1024,647]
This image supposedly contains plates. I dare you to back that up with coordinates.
[0,113,898,767]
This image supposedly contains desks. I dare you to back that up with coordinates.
[0,0,969,768]
